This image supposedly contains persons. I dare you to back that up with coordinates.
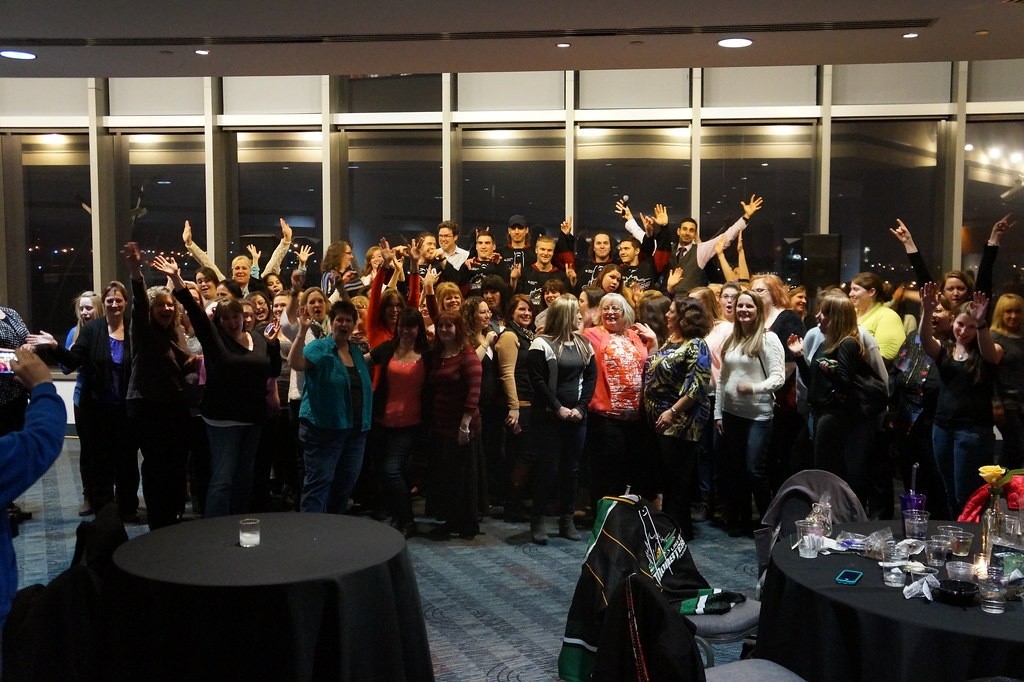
[0,306,67,627]
[61,181,1024,545]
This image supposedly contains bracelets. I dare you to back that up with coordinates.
[382,263,391,271]
[988,240,1000,246]
[296,334,305,340]
[671,407,679,416]
[460,426,470,433]
[976,320,987,330]
[410,268,420,274]
[439,257,446,262]
[480,344,487,351]
[742,216,750,225]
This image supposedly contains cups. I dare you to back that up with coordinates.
[922,539,951,566]
[881,542,911,588]
[907,566,939,596]
[945,561,974,582]
[899,494,927,537]
[931,535,957,558]
[937,525,964,535]
[238,518,261,547]
[974,516,1024,614]
[902,509,930,541]
[948,531,974,556]
[795,520,822,558]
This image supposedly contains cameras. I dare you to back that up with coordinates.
[0,349,19,376]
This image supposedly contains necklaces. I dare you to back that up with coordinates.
[954,348,967,359]
[441,345,459,365]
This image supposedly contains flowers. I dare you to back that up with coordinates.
[978,464,1008,510]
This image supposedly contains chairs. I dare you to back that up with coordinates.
[601,470,868,682]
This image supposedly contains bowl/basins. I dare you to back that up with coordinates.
[935,579,981,600]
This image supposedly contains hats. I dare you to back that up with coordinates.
[508,215,529,227]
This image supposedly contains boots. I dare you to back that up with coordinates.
[530,512,550,544]
[557,513,582,542]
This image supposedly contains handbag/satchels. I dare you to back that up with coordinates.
[855,362,889,416]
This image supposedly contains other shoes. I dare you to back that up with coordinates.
[690,500,710,522]
[125,517,140,523]
[573,516,595,529]
[430,525,479,541]
[396,522,417,539]
[375,509,392,520]
[504,503,532,522]
[483,507,497,515]
[7,502,32,520]
[79,508,94,516]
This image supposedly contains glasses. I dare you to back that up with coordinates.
[752,289,769,292]
[344,251,352,254]
[438,234,453,238]
[723,295,736,299]
[603,305,623,313]
[385,303,402,308]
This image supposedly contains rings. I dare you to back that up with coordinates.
[509,419,512,420]
[493,332,495,334]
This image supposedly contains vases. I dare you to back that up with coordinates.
[982,496,1006,555]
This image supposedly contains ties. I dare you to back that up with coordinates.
[677,247,687,262]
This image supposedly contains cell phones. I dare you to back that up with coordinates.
[835,569,863,585]
[510,422,522,435]
[266,324,274,335]
[816,357,838,368]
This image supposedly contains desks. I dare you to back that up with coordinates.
[111,512,435,682]
[754,521,1024,682]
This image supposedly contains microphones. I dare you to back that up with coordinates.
[622,195,629,215]
